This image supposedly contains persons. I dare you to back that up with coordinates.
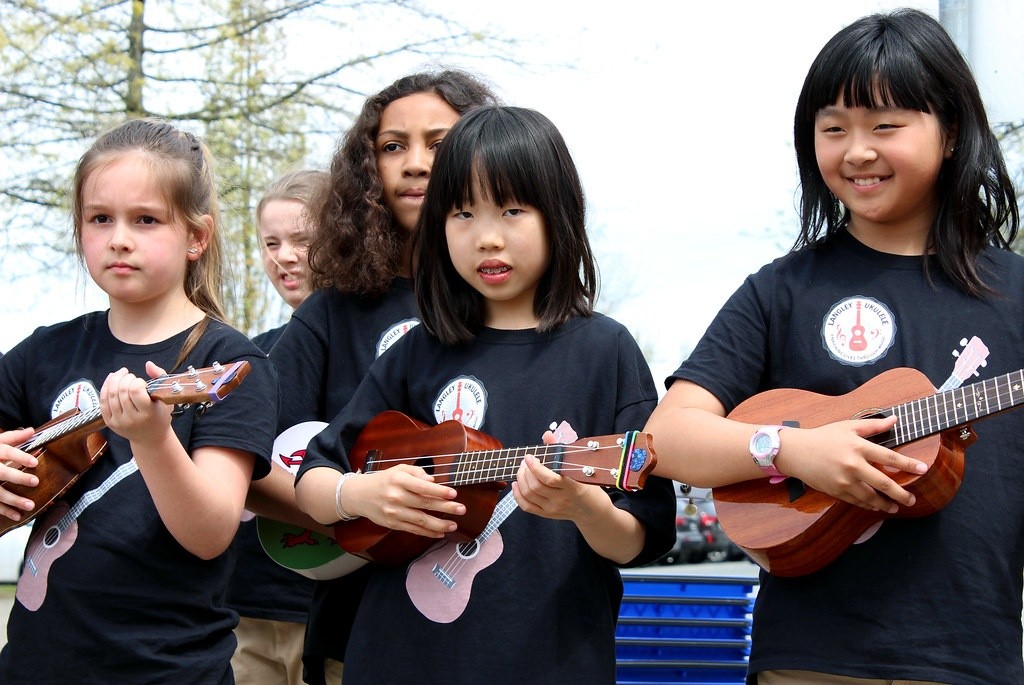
[224,171,346,684]
[295,107,677,685]
[247,69,498,685]
[643,8,1024,685]
[0,120,282,685]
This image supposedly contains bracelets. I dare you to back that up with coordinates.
[334,472,362,521]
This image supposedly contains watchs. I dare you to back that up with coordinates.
[748,424,788,484]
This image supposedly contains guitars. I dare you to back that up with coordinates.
[711,366,1024,578]
[331,408,658,568]
[0,361,253,540]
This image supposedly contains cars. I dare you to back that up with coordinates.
[665,491,745,567]
